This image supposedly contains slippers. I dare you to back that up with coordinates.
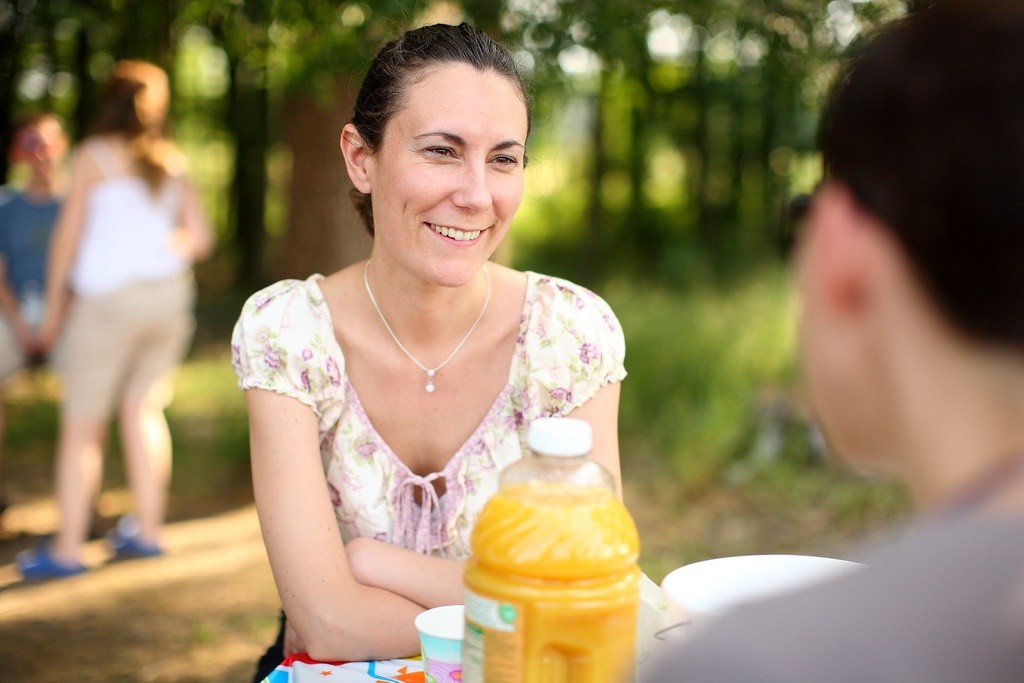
[106,523,161,560]
[17,542,85,579]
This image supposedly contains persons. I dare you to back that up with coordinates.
[230,22,625,683]
[0,59,217,584]
[647,0,1024,683]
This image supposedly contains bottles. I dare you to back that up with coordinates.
[461,417,640,683]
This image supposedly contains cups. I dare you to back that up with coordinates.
[414,605,464,683]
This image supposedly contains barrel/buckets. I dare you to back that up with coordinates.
[652,555,866,658]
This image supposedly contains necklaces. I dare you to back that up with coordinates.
[364,258,493,394]
